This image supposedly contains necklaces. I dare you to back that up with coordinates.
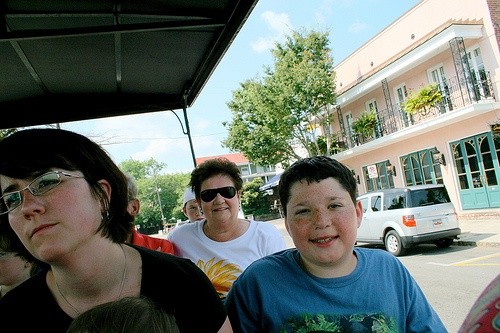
[54,241,127,316]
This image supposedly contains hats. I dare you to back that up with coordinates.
[182,185,197,209]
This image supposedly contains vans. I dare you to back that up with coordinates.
[356,184,462,256]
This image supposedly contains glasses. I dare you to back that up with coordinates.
[0,171,85,217]
[197,186,240,203]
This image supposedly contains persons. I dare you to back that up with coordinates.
[226,156,448,333]
[0,128,287,333]
